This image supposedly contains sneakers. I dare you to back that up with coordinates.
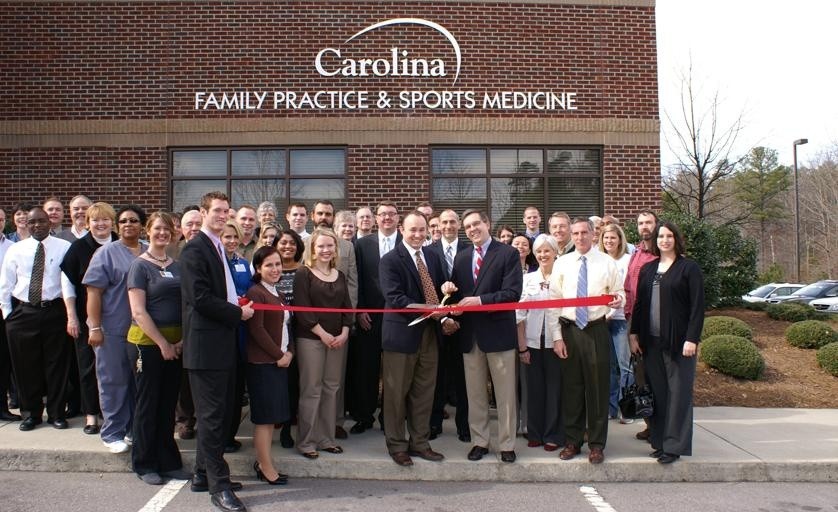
[620,415,634,423]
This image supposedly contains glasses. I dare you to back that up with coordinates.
[377,212,398,217]
[118,219,140,223]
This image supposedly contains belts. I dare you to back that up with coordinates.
[13,298,63,308]
[569,316,606,325]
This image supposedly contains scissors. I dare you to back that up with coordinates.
[406,293,462,327]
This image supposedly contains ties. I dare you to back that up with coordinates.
[474,247,482,284]
[383,238,390,255]
[445,246,453,279]
[219,244,238,305]
[415,251,439,305]
[576,257,588,329]
[28,242,45,306]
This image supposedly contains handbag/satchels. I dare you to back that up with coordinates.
[618,352,655,418]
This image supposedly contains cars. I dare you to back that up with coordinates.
[740,281,838,317]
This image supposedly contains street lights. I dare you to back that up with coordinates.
[791,138,812,283]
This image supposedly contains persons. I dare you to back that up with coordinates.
[2,192,704,511]
